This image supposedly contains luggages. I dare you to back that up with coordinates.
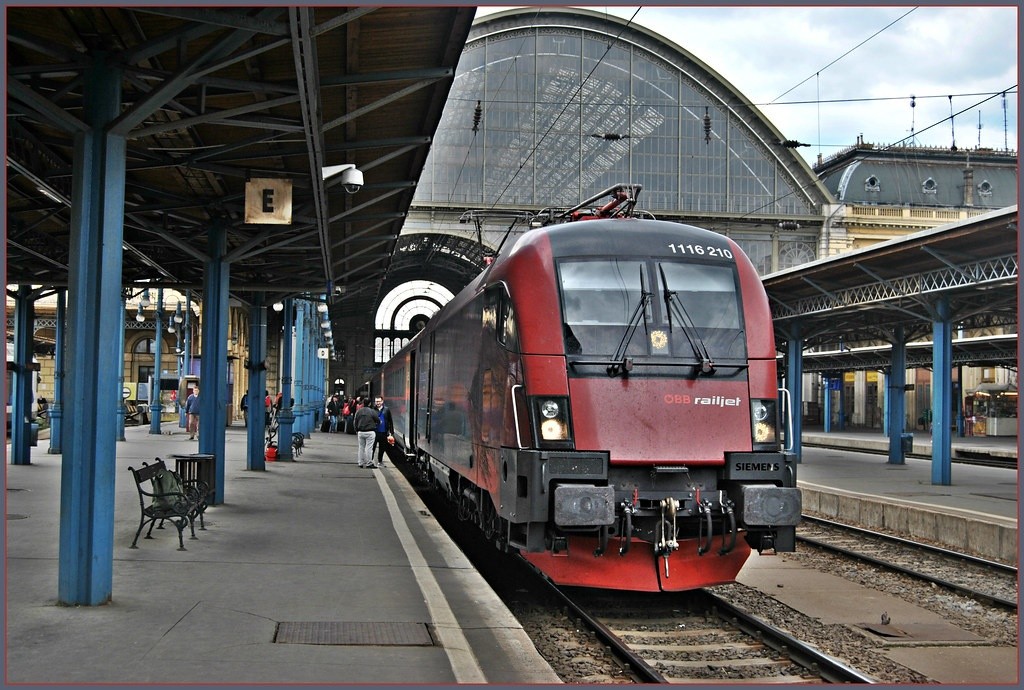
[337,418,345,432]
[346,416,357,435]
[320,415,331,433]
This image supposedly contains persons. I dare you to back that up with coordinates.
[323,393,371,436]
[184,385,200,440]
[37,395,48,418]
[353,397,381,469]
[240,389,248,426]
[371,395,395,467]
[265,390,272,429]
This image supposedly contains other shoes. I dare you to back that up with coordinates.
[189,437,194,440]
[245,423,247,428]
[377,462,384,467]
[358,464,364,468]
[365,463,378,469]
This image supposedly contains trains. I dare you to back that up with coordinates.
[368,182,802,593]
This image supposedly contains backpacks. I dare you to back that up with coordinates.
[265,396,270,407]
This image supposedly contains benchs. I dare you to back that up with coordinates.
[128,457,210,552]
[292,432,304,457]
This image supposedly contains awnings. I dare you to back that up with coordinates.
[964,382,1018,394]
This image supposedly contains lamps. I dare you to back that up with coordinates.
[318,302,336,360]
